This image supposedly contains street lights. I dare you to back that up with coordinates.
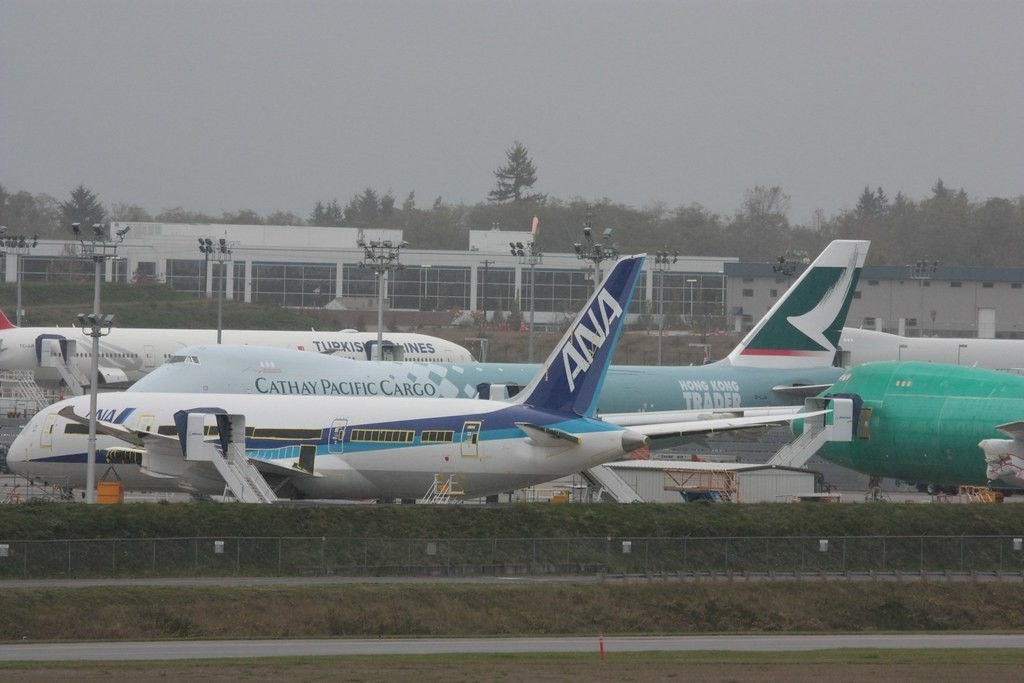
[509,240,544,363]
[357,239,410,361]
[775,248,804,289]
[0,233,40,327]
[654,248,681,367]
[904,253,941,335]
[70,222,130,504]
[199,238,234,344]
[574,225,620,289]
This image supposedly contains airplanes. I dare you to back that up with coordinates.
[838,327,1024,377]
[123,240,872,414]
[6,253,649,504]
[766,361,1024,492]
[0,309,483,384]
